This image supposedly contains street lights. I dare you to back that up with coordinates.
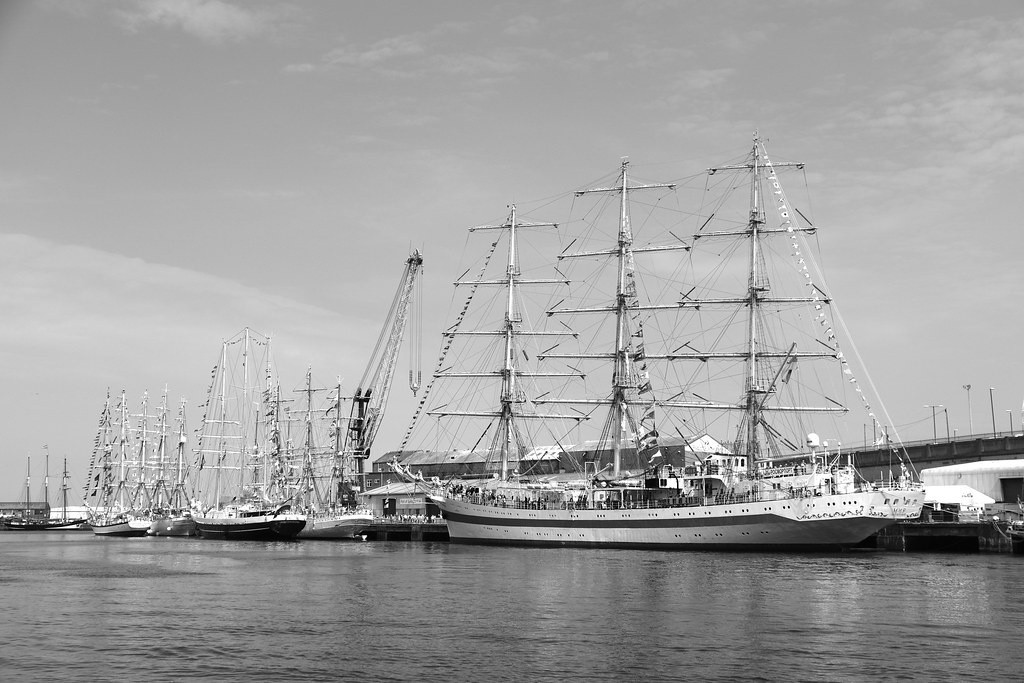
[924,404,945,442]
[990,388,997,438]
[954,428,958,442]
[1006,409,1014,435]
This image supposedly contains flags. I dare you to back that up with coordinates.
[648,451,662,464]
[94,474,100,481]
[626,248,643,337]
[640,372,649,379]
[390,242,499,464]
[637,431,659,454]
[91,491,97,497]
[95,483,99,487]
[762,156,909,478]
[633,352,645,362]
[200,456,206,470]
[639,401,656,421]
[638,380,652,395]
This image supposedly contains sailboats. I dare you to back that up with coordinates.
[0,129,926,547]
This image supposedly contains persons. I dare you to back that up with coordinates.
[378,513,442,523]
[569,495,587,510]
[453,483,503,507]
